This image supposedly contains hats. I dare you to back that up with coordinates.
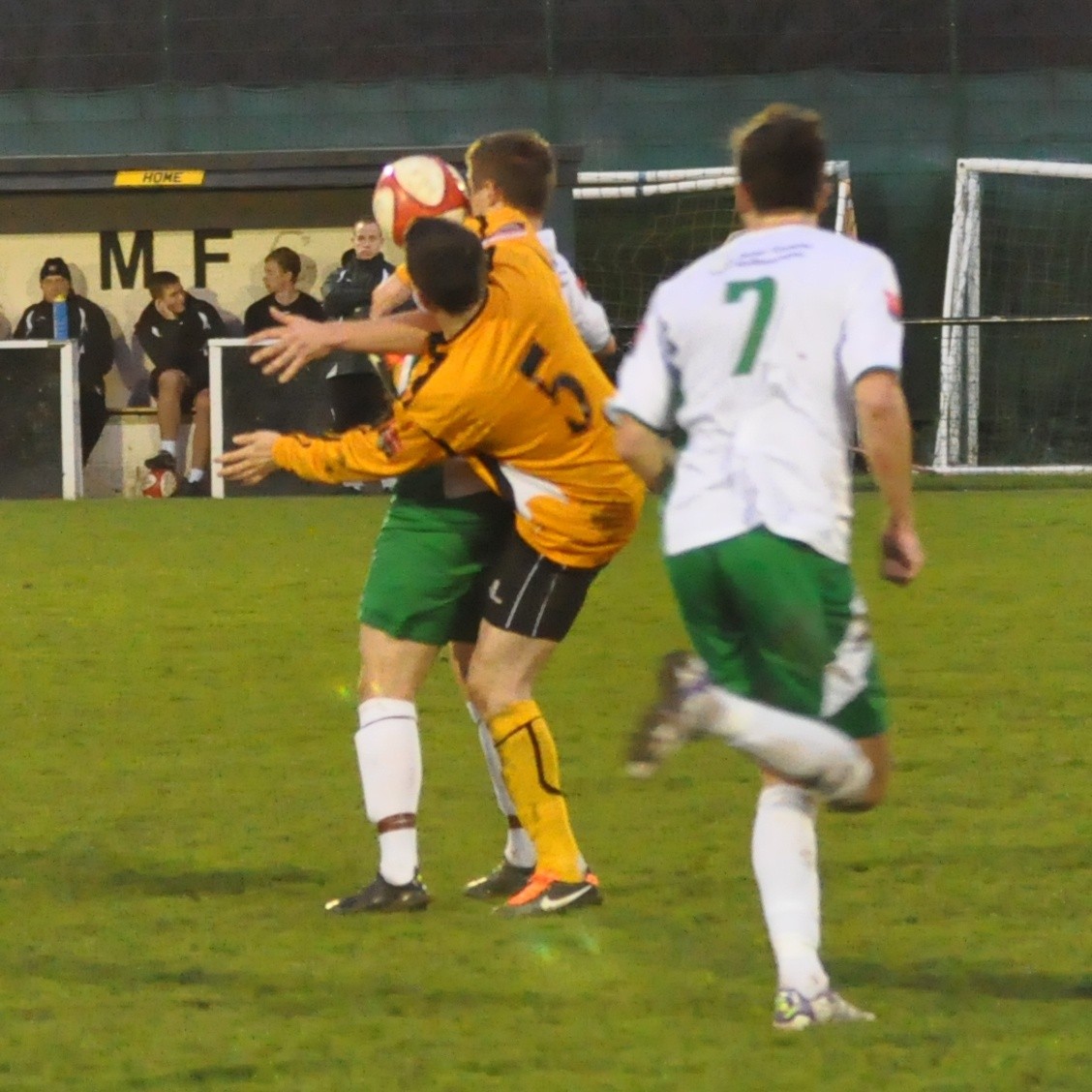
[41,258,71,283]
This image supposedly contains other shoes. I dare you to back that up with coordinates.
[171,477,199,497]
[380,477,398,493]
[342,482,363,492]
[145,450,177,470]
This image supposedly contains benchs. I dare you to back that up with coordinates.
[88,406,210,497]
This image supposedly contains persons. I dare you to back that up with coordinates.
[0,211,406,496]
[331,128,615,915]
[214,207,646,910]
[596,102,925,1045]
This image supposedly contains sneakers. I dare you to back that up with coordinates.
[325,869,427,915]
[771,988,877,1031]
[492,866,602,914]
[624,651,710,778]
[464,857,536,902]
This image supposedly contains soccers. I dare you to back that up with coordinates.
[370,153,468,249]
[141,468,178,499]
